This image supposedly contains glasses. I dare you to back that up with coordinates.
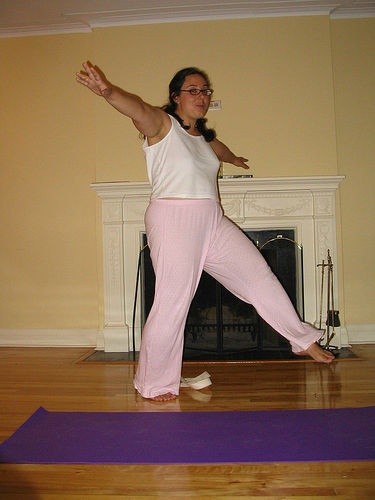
[179,88,214,96]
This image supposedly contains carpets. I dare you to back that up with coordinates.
[1,406,375,464]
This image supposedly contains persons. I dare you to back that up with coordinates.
[74,61,336,404]
